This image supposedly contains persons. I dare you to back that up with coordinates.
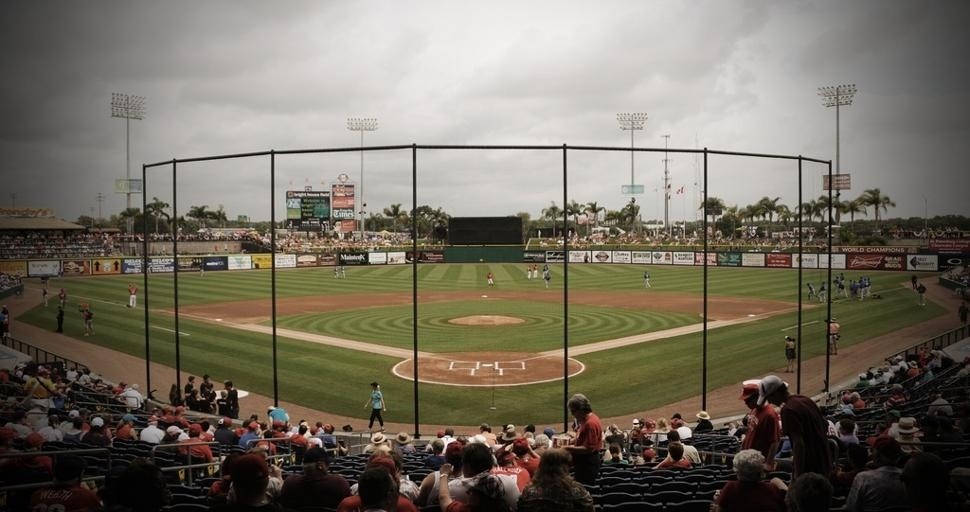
[2,216,969,509]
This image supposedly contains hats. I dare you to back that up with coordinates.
[366,431,413,474]
[55,454,88,481]
[757,374,784,407]
[96,378,140,392]
[738,379,761,400]
[216,412,353,464]
[170,373,234,404]
[842,350,968,511]
[0,402,209,449]
[370,382,379,388]
[830,318,838,322]
[432,424,555,452]
[11,362,90,381]
[602,410,712,459]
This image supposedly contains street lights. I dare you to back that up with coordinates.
[88,203,96,230]
[814,79,857,245]
[920,193,929,235]
[661,133,674,231]
[108,90,140,209]
[345,117,375,239]
[618,110,648,202]
[96,191,103,219]
[10,191,18,206]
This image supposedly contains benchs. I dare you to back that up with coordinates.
[0,229,118,300]
